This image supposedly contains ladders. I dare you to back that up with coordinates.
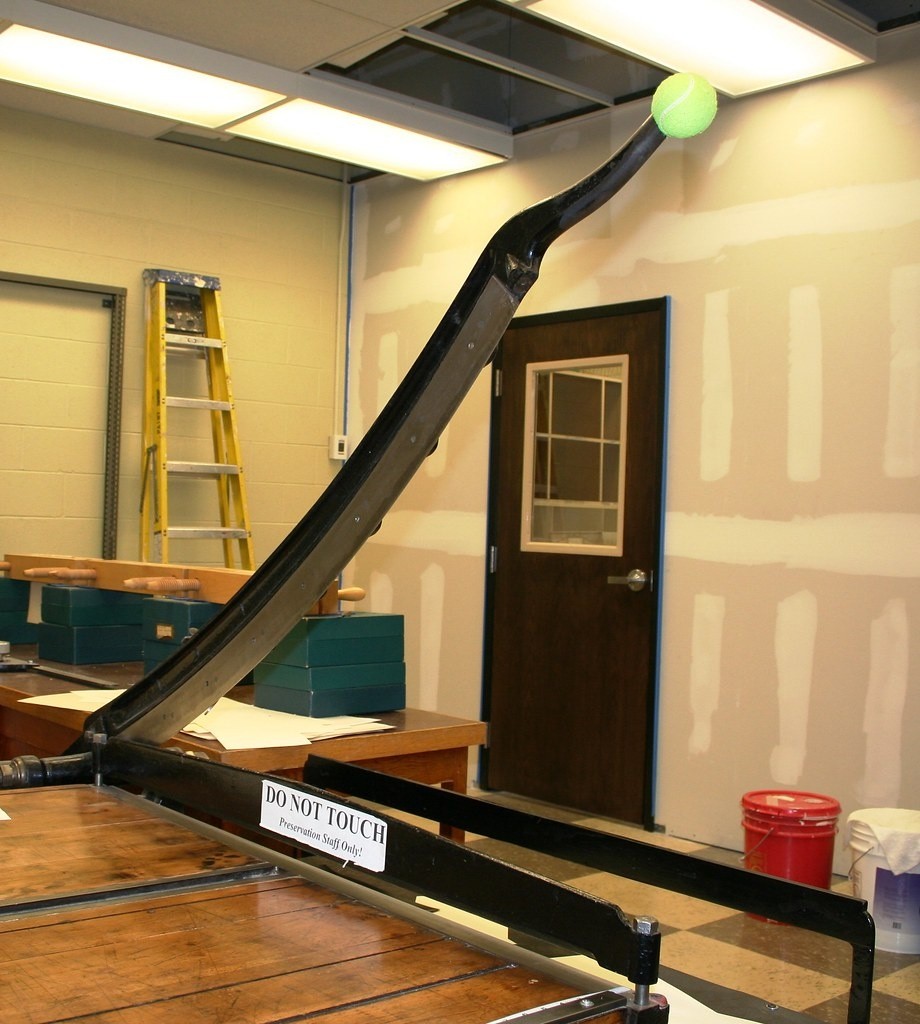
[131,260,264,578]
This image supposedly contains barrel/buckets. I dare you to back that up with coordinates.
[739,790,841,924]
[849,808,919,956]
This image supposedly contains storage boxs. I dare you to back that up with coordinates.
[0,578,38,643]
[254,610,405,719]
[142,597,254,685]
[37,585,153,665]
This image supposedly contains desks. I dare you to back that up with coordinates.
[0,643,488,859]
